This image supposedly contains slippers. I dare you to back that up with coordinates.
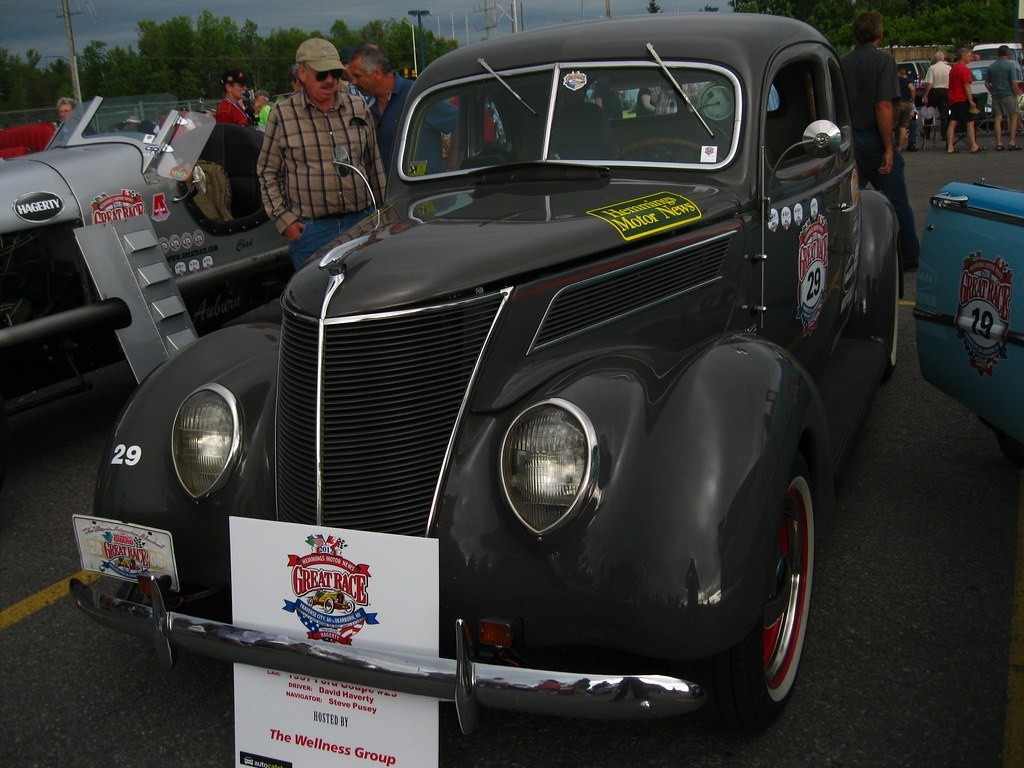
[947,148,960,154]
[971,145,988,153]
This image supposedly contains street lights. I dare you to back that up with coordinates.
[408,9,433,72]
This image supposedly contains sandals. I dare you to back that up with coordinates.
[996,144,1005,151]
[1007,144,1024,151]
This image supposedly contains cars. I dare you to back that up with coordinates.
[67,11,903,740]
[910,177,1024,474]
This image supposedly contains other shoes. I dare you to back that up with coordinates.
[942,136,947,141]
[920,132,929,140]
[906,145,918,152]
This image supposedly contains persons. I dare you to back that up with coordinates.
[347,46,459,198]
[837,12,921,299]
[440,79,721,171]
[53,95,91,138]
[894,45,1023,152]
[146,63,377,140]
[255,37,389,270]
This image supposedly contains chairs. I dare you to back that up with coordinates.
[0,146,33,159]
[521,104,615,162]
[919,92,991,151]
[172,124,267,221]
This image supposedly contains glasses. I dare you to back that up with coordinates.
[302,63,343,81]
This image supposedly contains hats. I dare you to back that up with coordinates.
[254,90,270,99]
[221,70,254,85]
[296,38,347,71]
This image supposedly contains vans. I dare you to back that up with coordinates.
[966,60,1024,130]
[898,59,935,104]
[971,43,1024,64]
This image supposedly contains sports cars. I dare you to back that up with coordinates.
[0,92,299,418]
